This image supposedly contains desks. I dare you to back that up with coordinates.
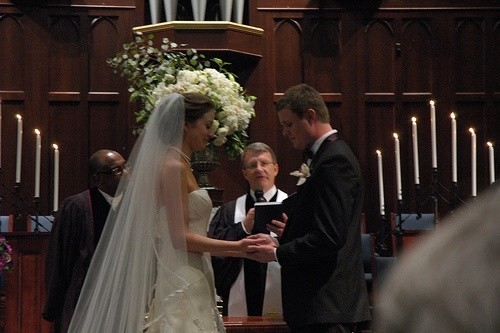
[219,316,289,333]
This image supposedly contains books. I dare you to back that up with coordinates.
[254,191,298,230]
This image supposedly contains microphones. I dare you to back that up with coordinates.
[254,188,267,202]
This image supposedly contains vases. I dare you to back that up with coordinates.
[0,290,8,332]
[191,140,217,187]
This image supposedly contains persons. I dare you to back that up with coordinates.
[141,92,266,333]
[206,141,310,316]
[244,84,371,333]
[40,149,131,332]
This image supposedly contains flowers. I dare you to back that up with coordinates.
[289,163,311,187]
[0,237,14,275]
[106,32,258,160]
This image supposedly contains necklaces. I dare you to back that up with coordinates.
[162,142,197,172]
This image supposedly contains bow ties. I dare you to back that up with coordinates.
[302,151,313,163]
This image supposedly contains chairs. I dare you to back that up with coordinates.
[391,212,439,258]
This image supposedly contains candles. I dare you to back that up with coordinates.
[412,117,419,184]
[35,129,41,197]
[53,144,58,212]
[377,150,385,219]
[430,100,437,168]
[450,112,457,182]
[16,115,22,182]
[393,132,402,200]
[470,128,477,196]
[487,141,494,184]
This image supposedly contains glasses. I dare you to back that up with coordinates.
[96,162,129,176]
